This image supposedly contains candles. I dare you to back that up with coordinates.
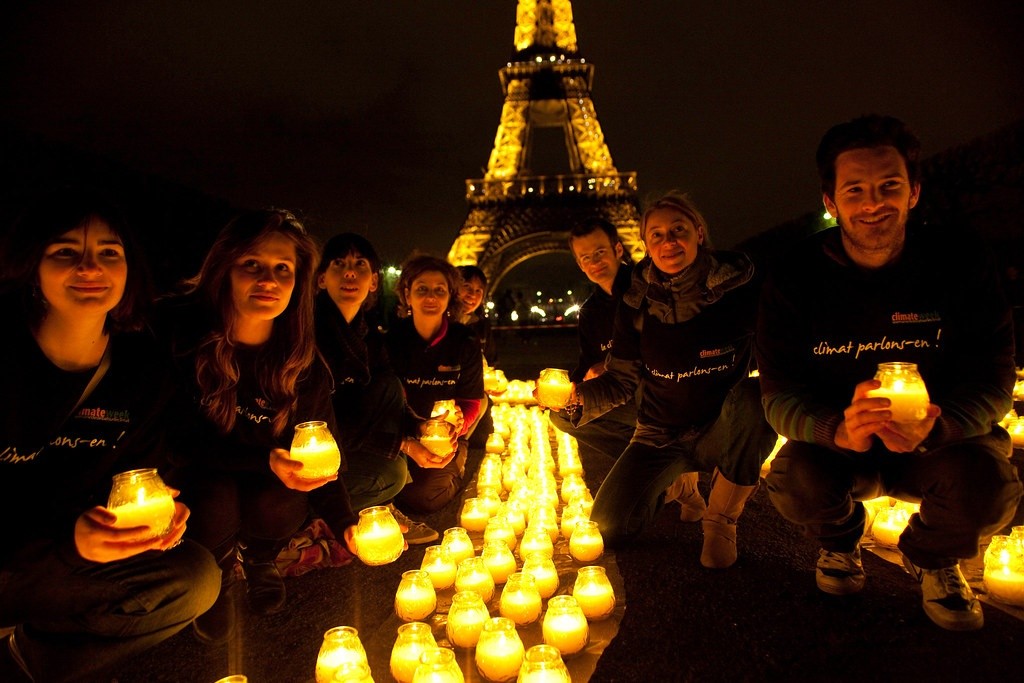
[291,421,340,479]
[870,361,929,423]
[864,495,920,548]
[999,369,1024,449]
[982,524,1024,608]
[313,363,616,682]
[107,469,174,536]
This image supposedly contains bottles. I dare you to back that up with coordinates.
[868,362,929,423]
[107,468,176,543]
[862,496,923,548]
[315,367,615,682]
[290,420,341,482]
[996,366,1024,449]
[984,525,1023,604]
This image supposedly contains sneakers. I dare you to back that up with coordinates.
[384,501,439,544]
[814,542,866,593]
[903,549,985,631]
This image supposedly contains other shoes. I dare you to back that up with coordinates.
[237,556,287,614]
[190,565,237,647]
[453,441,468,472]
[309,519,354,568]
[8,632,34,682]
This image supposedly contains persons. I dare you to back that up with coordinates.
[456,266,487,319]
[158,207,360,641]
[532,195,778,571]
[757,116,1024,630]
[384,250,486,514]
[0,181,223,682]
[311,234,440,544]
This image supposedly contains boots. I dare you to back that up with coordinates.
[664,470,708,523]
[699,467,762,566]
